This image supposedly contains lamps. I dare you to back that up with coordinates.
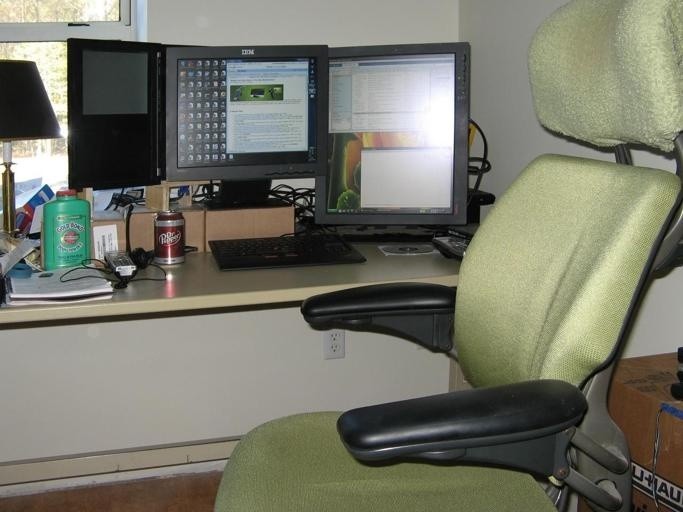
[0,58,61,240]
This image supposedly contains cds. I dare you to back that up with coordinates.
[381,244,435,255]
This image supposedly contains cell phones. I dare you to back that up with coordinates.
[433,236,466,258]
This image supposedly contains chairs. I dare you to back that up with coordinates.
[213,3,682,512]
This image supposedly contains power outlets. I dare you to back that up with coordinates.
[323,329,345,359]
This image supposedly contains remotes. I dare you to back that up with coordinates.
[103,250,139,281]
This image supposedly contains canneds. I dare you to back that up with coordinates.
[154,211,185,265]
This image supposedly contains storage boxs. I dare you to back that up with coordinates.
[577,348,682,512]
[89,202,294,266]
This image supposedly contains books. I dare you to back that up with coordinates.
[5,263,114,307]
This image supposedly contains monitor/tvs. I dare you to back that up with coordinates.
[162,44,329,210]
[313,41,471,242]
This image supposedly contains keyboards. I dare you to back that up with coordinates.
[208,232,366,272]
